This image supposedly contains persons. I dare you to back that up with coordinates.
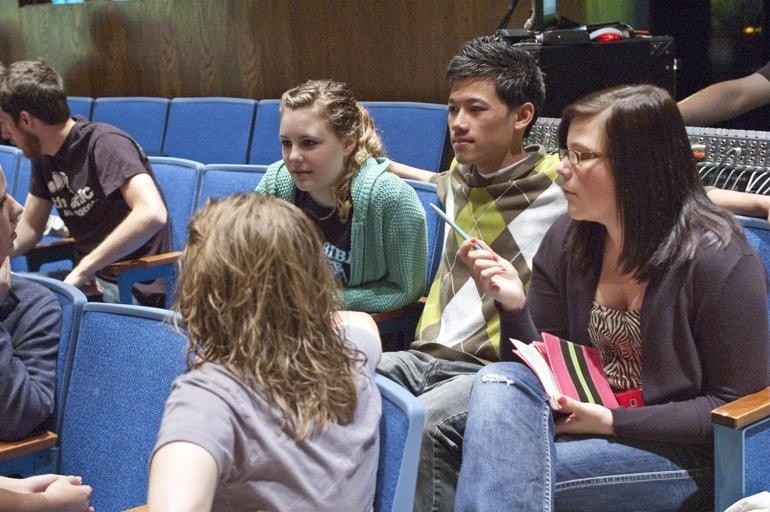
[0,60,173,305]
[0,472,98,512]
[376,35,769,512]
[415,83,770,511]
[144,193,384,512]
[0,166,61,441]
[676,60,770,128]
[256,77,427,311]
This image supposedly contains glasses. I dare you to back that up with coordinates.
[557,147,609,167]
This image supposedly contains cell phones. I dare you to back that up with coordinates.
[614,389,645,408]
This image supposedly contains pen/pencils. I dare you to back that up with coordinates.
[429,203,483,249]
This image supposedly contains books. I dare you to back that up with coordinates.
[508,329,620,413]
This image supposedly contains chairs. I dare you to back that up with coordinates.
[0,85,770,512]
